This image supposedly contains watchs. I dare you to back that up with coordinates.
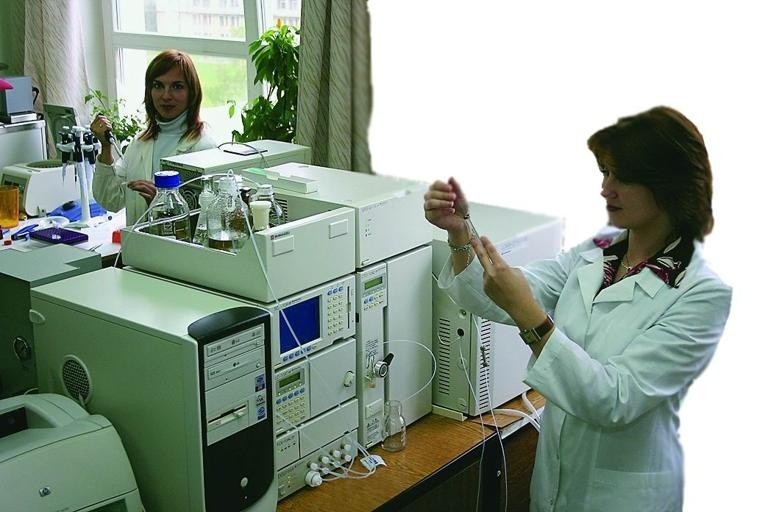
[519,315,555,345]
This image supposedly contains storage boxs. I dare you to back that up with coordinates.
[121,207,355,303]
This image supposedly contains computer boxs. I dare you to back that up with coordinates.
[159,138,313,211]
[29,266,279,512]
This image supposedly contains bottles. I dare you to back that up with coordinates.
[382,400,406,451]
[148,170,285,252]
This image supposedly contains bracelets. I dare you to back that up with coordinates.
[448,239,472,254]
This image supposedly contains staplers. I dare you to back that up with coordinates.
[12,224,39,240]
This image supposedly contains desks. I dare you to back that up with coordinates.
[277,387,549,512]
[1,209,124,270]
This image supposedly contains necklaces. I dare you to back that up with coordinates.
[621,260,634,272]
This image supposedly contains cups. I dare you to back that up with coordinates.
[0,186,19,229]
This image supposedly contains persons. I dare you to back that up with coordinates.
[90,50,219,227]
[424,105,733,512]
